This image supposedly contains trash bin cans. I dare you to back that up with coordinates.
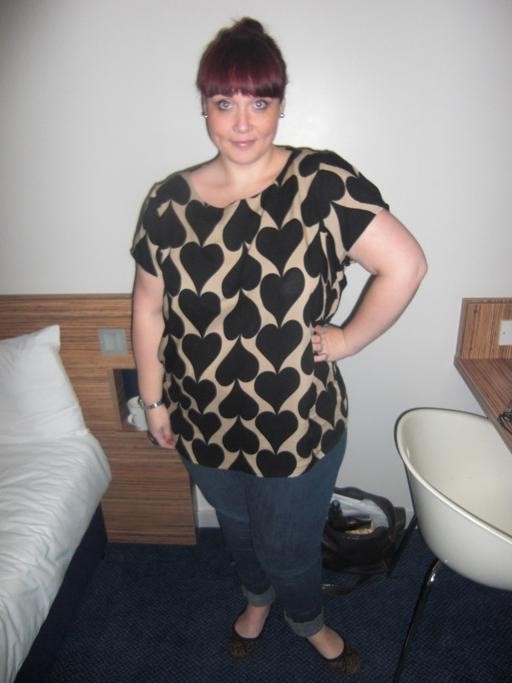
[322,487,396,594]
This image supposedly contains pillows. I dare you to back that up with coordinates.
[1,322,91,445]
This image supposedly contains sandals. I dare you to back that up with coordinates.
[302,622,361,678]
[228,598,276,660]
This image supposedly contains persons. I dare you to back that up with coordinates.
[126,15,429,677]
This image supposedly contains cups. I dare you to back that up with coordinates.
[126,395,149,432]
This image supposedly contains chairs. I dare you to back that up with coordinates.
[385,405,511,682]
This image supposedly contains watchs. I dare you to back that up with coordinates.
[137,397,164,411]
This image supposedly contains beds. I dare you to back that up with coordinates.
[2,292,200,681]
